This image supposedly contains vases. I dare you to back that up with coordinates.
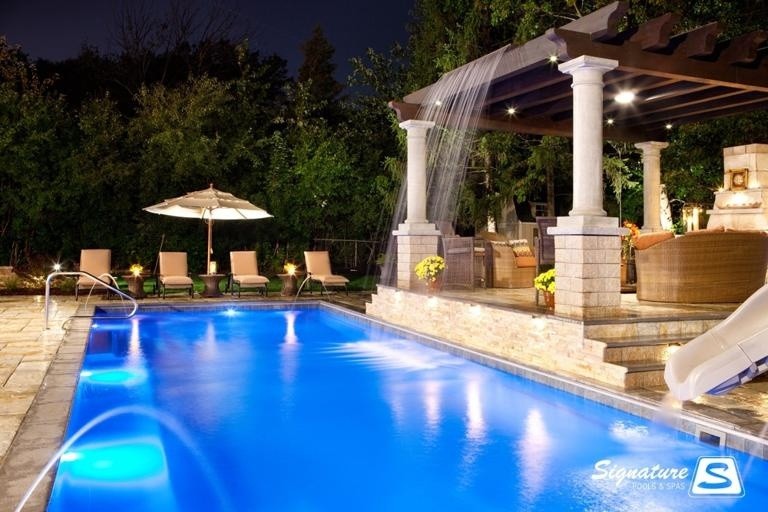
[428,277,441,290]
[543,292,554,307]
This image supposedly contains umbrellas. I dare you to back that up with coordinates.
[141,183,275,276]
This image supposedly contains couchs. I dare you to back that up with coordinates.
[489,239,536,288]
[634,226,768,303]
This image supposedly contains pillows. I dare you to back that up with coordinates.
[761,231,768,237]
[685,225,725,235]
[726,227,760,234]
[634,231,674,251]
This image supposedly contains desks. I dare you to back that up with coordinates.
[198,273,226,297]
[122,274,151,299]
[277,273,304,297]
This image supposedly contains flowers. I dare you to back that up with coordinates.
[533,269,557,294]
[416,256,448,285]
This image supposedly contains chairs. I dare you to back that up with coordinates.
[77,248,112,299]
[304,250,350,296]
[228,251,270,298]
[157,251,194,299]
[535,217,557,305]
[436,221,486,292]
